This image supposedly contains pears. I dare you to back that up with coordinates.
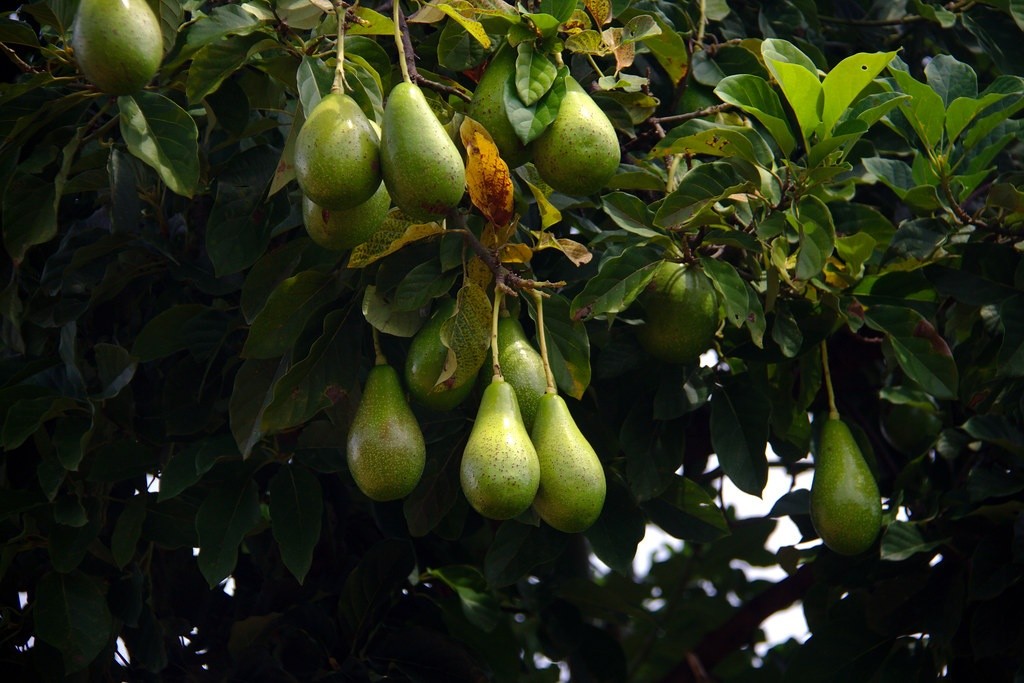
[75,0,883,547]
